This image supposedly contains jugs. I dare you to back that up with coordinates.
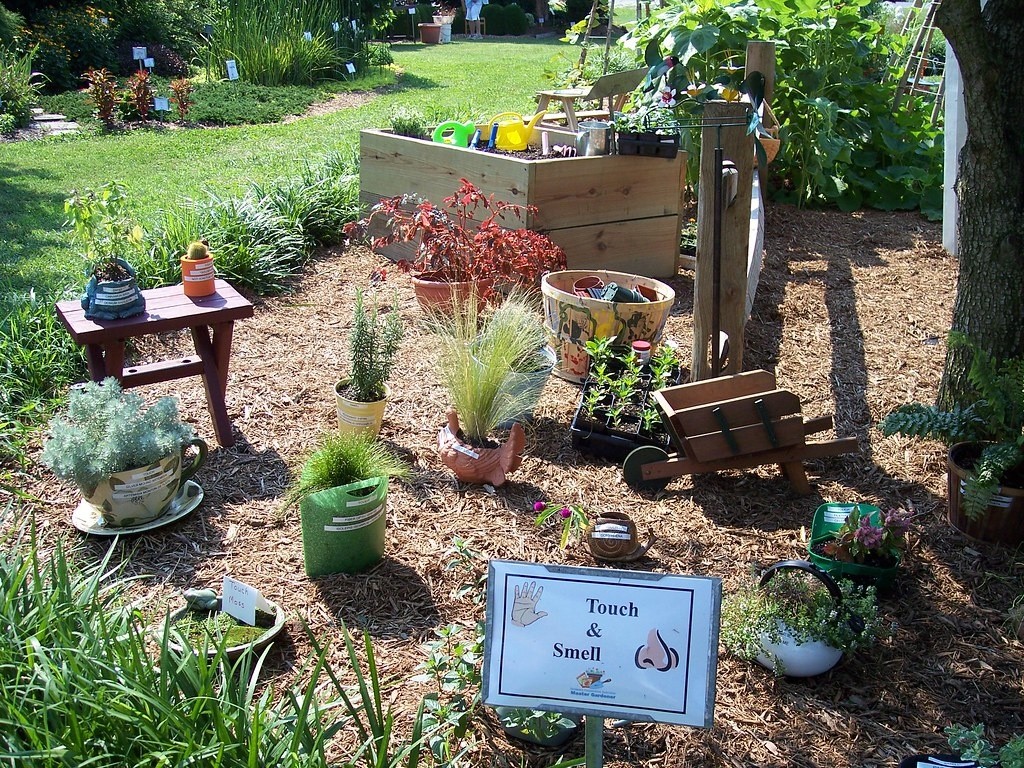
[488,110,546,151]
[433,120,475,147]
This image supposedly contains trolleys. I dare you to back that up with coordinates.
[623,369,859,494]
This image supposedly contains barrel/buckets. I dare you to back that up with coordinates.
[573,276,658,304]
[433,16,454,44]
[541,270,676,385]
[418,23,441,42]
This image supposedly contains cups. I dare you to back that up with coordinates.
[575,122,611,156]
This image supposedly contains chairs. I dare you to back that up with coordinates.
[532,67,651,132]
[461,0,486,35]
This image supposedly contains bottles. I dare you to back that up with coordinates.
[632,341,651,365]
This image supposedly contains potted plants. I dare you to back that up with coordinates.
[43,376,209,535]
[410,272,693,488]
[332,285,407,442]
[569,335,684,459]
[419,537,581,764]
[181,242,216,296]
[157,595,285,662]
[390,107,427,140]
[284,429,415,579]
[343,175,569,315]
[59,181,146,319]
[713,334,1024,767]
[430,7,456,43]
[615,114,680,158]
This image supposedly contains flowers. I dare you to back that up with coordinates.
[532,501,598,548]
[823,501,915,566]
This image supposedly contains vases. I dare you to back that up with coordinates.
[806,502,900,577]
[580,511,660,561]
[419,23,441,43]
[541,270,675,385]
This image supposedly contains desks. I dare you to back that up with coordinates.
[56,278,254,447]
